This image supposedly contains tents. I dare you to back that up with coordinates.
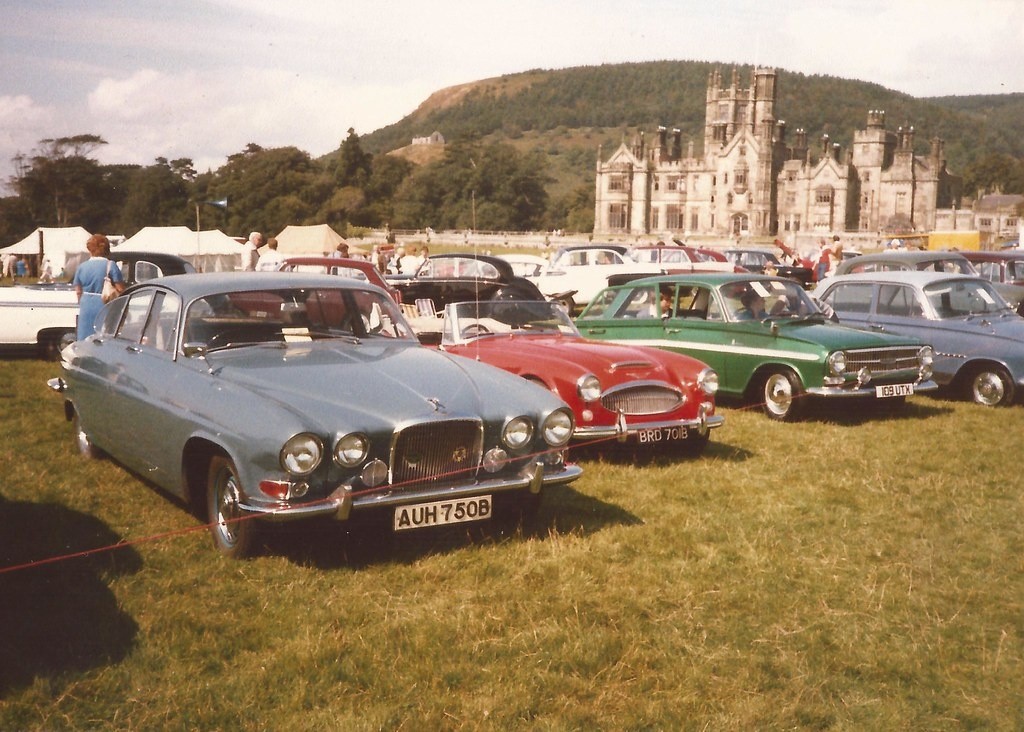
[240,232,263,272]
[0,225,113,283]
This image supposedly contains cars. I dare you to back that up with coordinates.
[779,271,1024,408]
[15,254,215,357]
[572,272,940,422]
[352,253,552,331]
[539,246,667,317]
[826,251,1024,316]
[46,271,583,561]
[228,257,402,327]
[463,255,552,288]
[665,248,751,272]
[722,250,813,291]
[417,300,725,457]
[917,251,1024,287]
[624,246,736,275]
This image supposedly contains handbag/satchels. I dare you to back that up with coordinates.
[102,260,121,305]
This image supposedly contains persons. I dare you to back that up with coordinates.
[504,235,508,244]
[740,233,845,323]
[109,234,124,248]
[73,232,126,345]
[254,237,285,272]
[636,285,674,321]
[3,252,65,281]
[604,251,613,264]
[890,239,927,251]
[540,227,575,267]
[321,220,494,279]
[110,226,245,274]
